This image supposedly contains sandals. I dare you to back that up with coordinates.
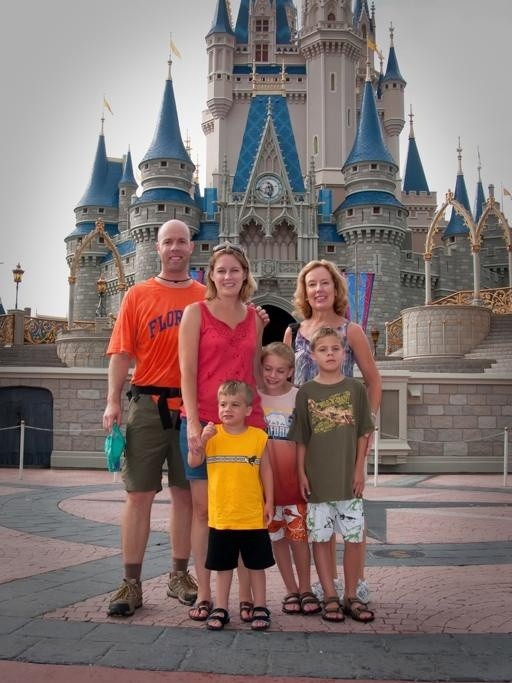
[189,579,376,633]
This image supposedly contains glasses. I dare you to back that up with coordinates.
[213,244,242,252]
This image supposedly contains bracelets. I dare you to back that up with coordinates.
[371,411,379,431]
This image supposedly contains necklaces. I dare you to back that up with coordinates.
[156,274,192,285]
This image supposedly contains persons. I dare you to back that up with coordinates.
[257,340,320,613]
[178,241,268,620]
[102,218,270,619]
[186,378,274,630]
[282,259,383,606]
[287,325,380,622]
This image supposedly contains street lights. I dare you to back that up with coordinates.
[12,261,23,309]
[370,324,379,355]
[97,273,107,316]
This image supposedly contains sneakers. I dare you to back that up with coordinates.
[106,576,141,618]
[166,572,198,606]
